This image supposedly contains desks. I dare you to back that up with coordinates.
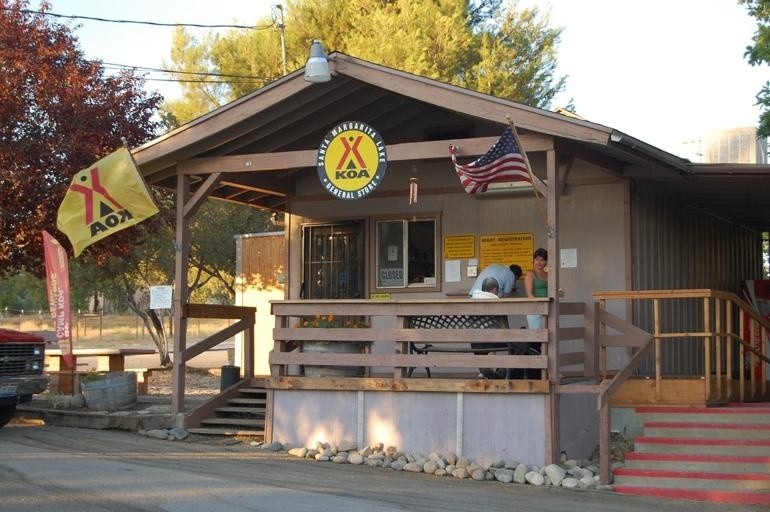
[44,347,156,397]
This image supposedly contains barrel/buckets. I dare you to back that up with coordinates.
[80,372,137,412]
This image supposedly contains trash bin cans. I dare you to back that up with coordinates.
[221,365,240,393]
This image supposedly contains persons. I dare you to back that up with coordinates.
[468,276,511,349]
[468,262,523,380]
[523,248,569,379]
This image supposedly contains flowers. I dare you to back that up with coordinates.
[292,311,374,348]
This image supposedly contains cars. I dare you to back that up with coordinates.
[0,329,48,428]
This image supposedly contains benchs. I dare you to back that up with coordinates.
[405,315,530,380]
[46,368,157,397]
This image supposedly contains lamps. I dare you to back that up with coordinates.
[303,38,335,83]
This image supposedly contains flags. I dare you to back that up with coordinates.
[56,146,160,259]
[448,126,533,195]
